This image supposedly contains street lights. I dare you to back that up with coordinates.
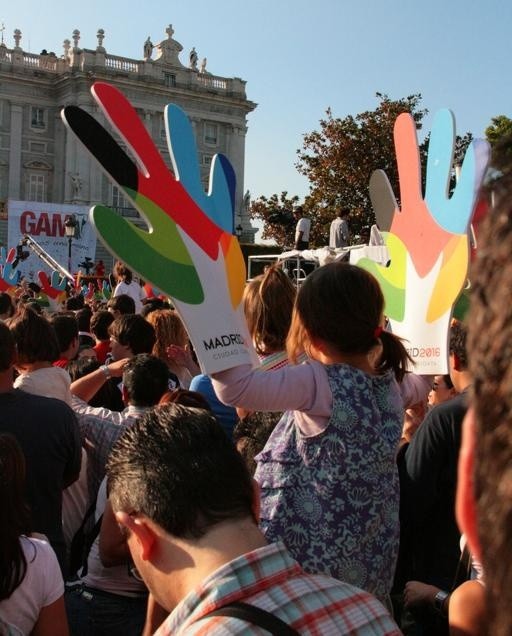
[235,225,243,241]
[63,217,78,274]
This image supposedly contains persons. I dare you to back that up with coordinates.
[1,202,512,635]
